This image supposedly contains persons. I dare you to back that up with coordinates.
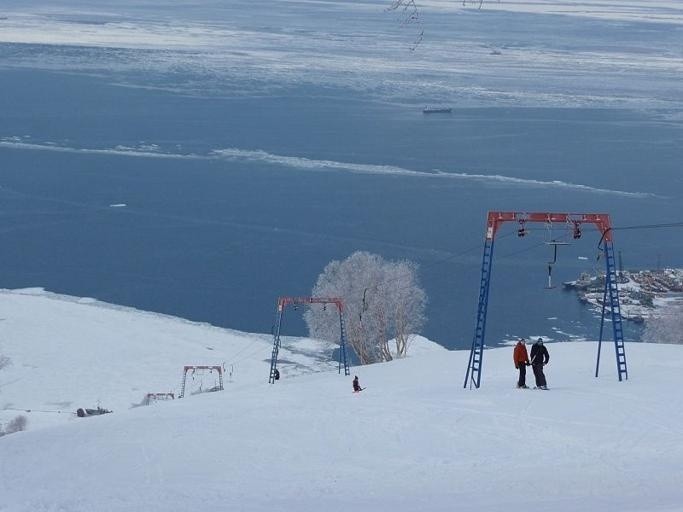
[353,376,361,391]
[530,338,550,390]
[514,338,529,388]
[274,369,279,379]
